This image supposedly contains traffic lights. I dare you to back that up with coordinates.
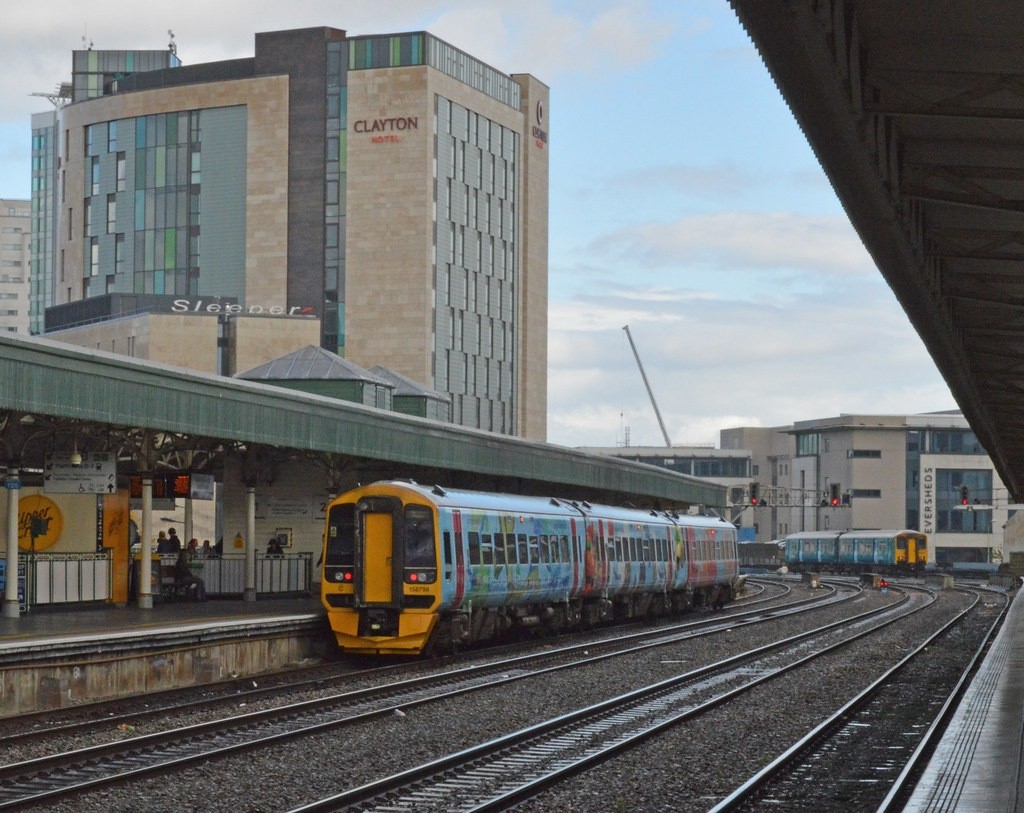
[829,483,841,508]
[749,483,760,508]
[960,485,969,505]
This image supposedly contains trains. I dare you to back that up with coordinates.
[738,529,928,576]
[321,479,741,654]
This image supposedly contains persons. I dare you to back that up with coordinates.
[187,539,198,553]
[174,550,207,603]
[155,527,184,565]
[199,540,212,553]
[265,538,285,559]
[316,534,324,567]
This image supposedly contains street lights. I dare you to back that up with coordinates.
[987,520,996,563]
